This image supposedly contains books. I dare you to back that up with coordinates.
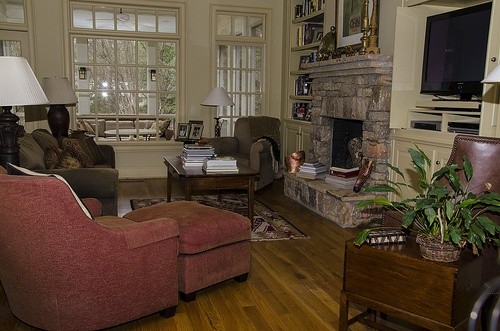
[178,145,239,175]
[290,1,328,47]
[297,159,360,190]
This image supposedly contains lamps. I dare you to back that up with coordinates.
[0,56,50,164]
[200,87,235,137]
[42,78,78,138]
[79,67,86,80]
[480,63,500,84]
[151,70,157,81]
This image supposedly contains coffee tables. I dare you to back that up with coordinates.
[163,156,260,231]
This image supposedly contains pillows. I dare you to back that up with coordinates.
[44,142,63,169]
[29,128,57,151]
[56,146,92,169]
[79,137,105,165]
[6,162,94,220]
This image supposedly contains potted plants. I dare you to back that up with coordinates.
[354,142,500,263]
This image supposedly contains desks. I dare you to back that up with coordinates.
[338,235,479,331]
[103,129,161,141]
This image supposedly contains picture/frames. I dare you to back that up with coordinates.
[335,0,373,50]
[189,124,204,139]
[177,123,189,138]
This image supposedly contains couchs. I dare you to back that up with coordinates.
[0,167,179,331]
[204,116,282,192]
[82,119,172,140]
[19,129,118,217]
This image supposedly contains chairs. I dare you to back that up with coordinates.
[381,135,500,228]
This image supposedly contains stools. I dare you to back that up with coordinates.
[124,201,251,303]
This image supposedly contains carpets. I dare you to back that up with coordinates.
[129,194,310,243]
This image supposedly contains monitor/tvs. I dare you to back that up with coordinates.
[420,2,492,101]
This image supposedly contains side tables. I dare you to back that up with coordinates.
[175,138,201,144]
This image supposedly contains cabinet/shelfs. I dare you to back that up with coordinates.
[389,128,452,205]
[284,9,324,159]
